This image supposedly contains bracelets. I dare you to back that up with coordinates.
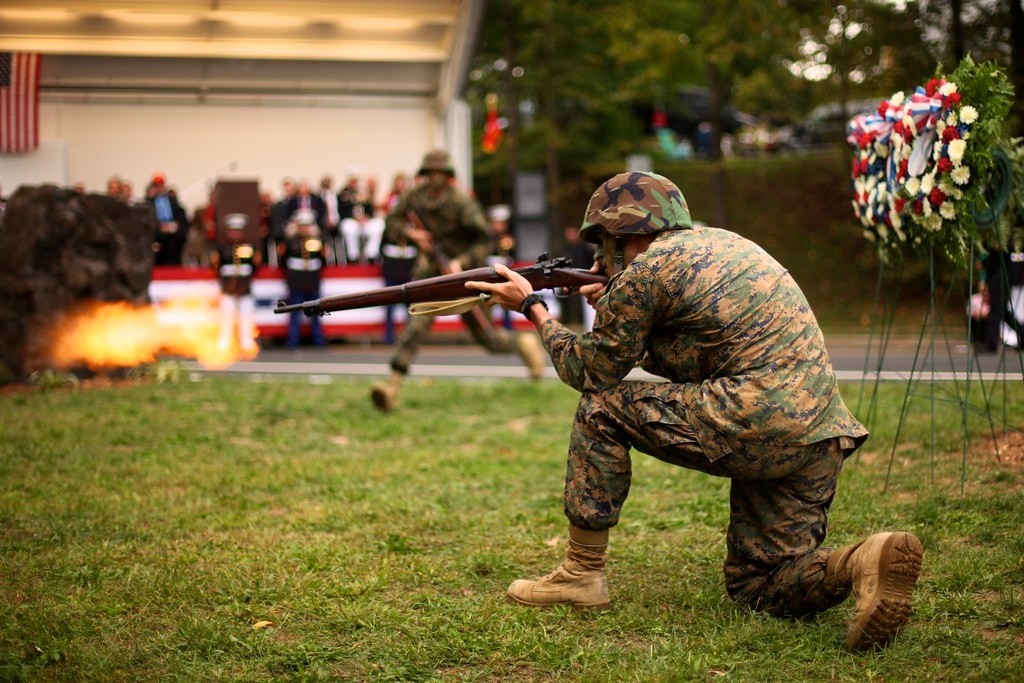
[520,294,549,321]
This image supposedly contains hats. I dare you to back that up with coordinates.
[294,212,315,224]
[152,177,165,187]
[222,211,248,230]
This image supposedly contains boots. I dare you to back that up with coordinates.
[822,531,923,653]
[506,538,611,611]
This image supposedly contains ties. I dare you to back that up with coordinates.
[299,237,311,261]
[232,240,243,266]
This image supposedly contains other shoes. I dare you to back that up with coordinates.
[520,333,545,379]
[368,381,398,413]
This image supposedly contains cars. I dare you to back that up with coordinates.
[642,81,890,160]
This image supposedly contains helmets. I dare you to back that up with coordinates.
[578,170,694,245]
[415,150,456,177]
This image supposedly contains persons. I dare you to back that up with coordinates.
[214,214,262,354]
[277,209,329,351]
[465,173,923,654]
[368,150,543,413]
[72,150,514,266]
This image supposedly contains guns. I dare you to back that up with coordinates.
[273,252,615,318]
